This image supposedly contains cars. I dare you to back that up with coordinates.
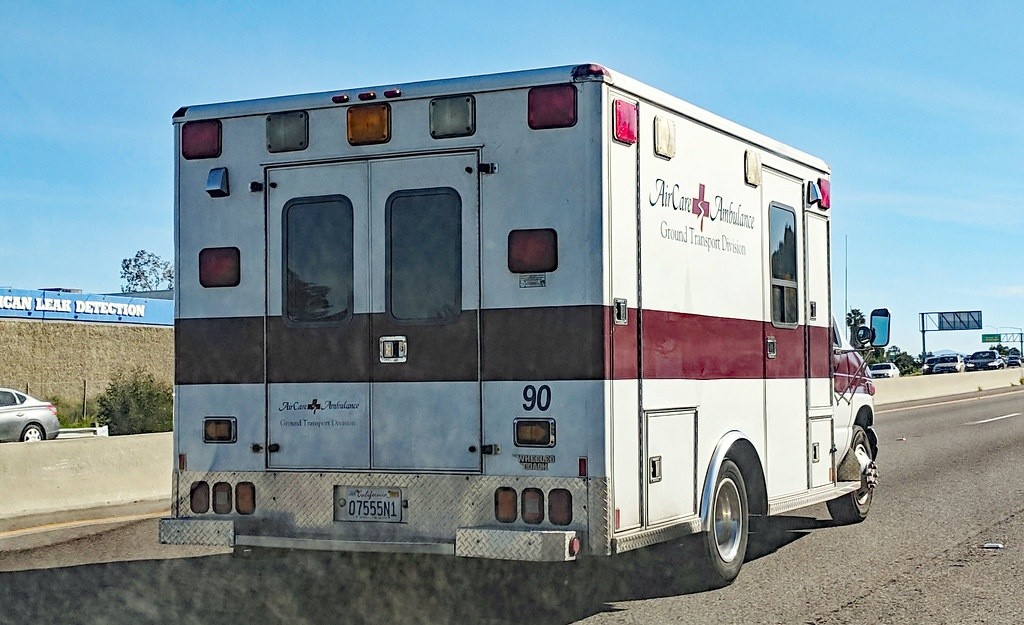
[0,388,61,441]
[870,363,900,378]
[923,354,965,375]
[963,350,1024,372]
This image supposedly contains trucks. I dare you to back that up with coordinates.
[157,64,890,588]
